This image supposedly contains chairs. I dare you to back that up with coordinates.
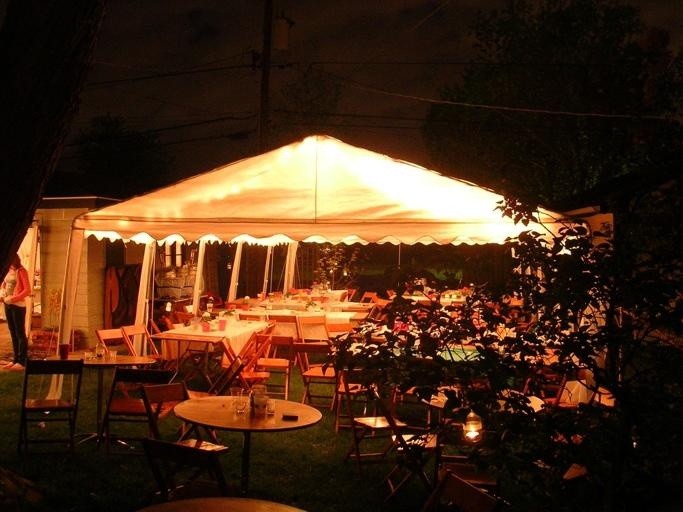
[439,469,508,512]
[370,390,442,498]
[19,357,83,448]
[140,381,230,501]
[96,365,179,463]
[96,284,602,435]
[339,368,409,469]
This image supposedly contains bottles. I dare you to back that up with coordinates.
[248,385,270,418]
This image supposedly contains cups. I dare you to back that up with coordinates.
[217,319,228,331]
[83,343,117,361]
[228,387,246,414]
[58,343,71,360]
[266,399,277,415]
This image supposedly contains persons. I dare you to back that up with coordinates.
[0,255,32,370]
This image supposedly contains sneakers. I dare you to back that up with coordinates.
[10,362,25,371]
[2,362,14,369]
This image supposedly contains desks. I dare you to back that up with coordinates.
[175,395,323,500]
[43,350,158,452]
